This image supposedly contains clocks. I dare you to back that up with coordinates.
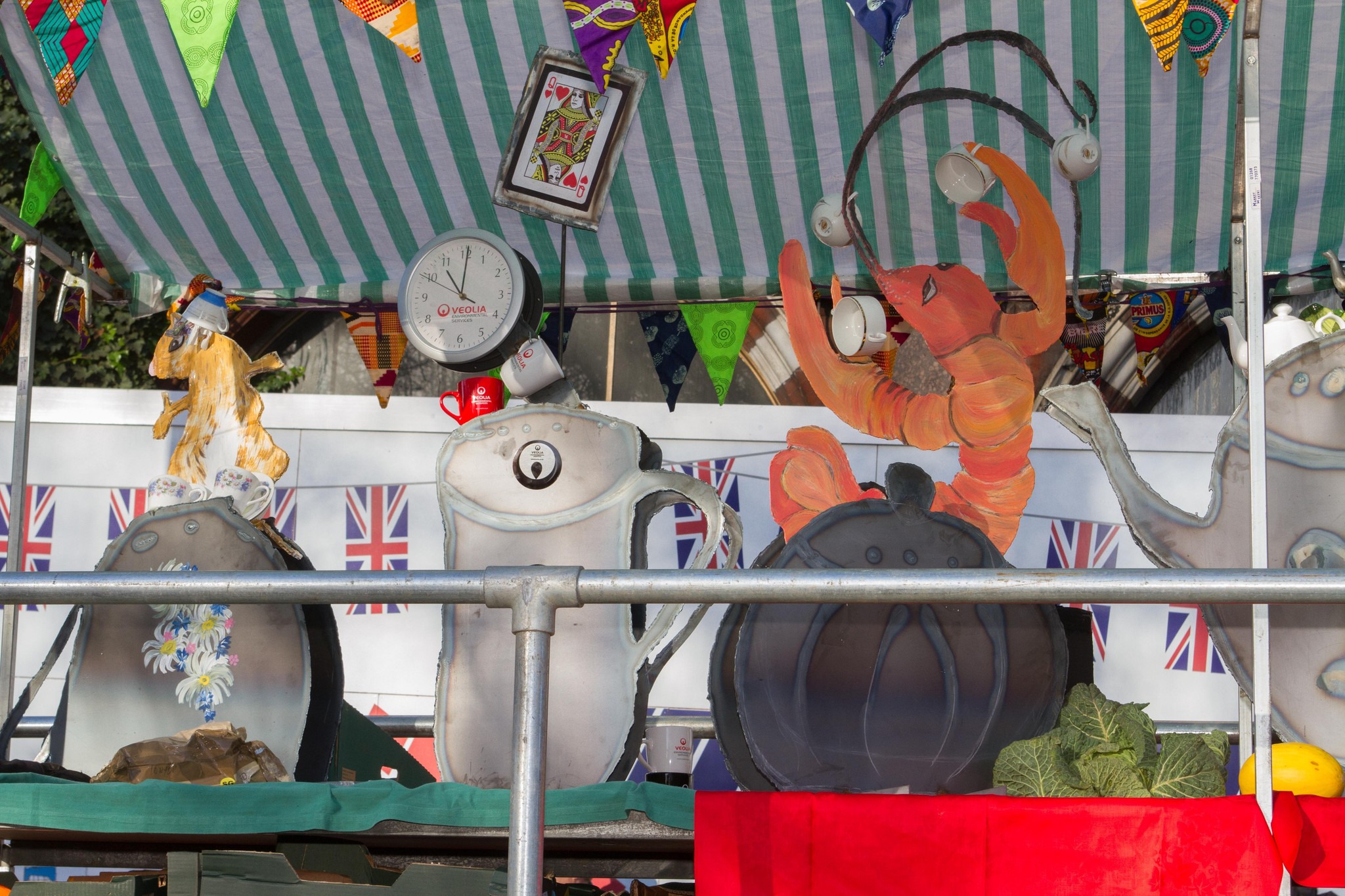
[398,227,525,364]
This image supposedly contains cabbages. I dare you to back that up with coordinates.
[989,688,1229,798]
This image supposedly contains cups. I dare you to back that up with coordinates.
[638,725,695,792]
[440,376,505,426]
[1054,113,1103,182]
[500,337,565,398]
[181,288,229,336]
[935,142,997,206]
[811,191,863,248]
[830,295,886,358]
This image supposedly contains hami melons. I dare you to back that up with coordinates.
[1238,742,1345,798]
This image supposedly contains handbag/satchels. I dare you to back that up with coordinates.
[91,721,293,786]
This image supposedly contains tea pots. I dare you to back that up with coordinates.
[1221,304,1345,371]
[1321,249,1345,293]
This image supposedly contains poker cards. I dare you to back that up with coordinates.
[489,45,649,232]
